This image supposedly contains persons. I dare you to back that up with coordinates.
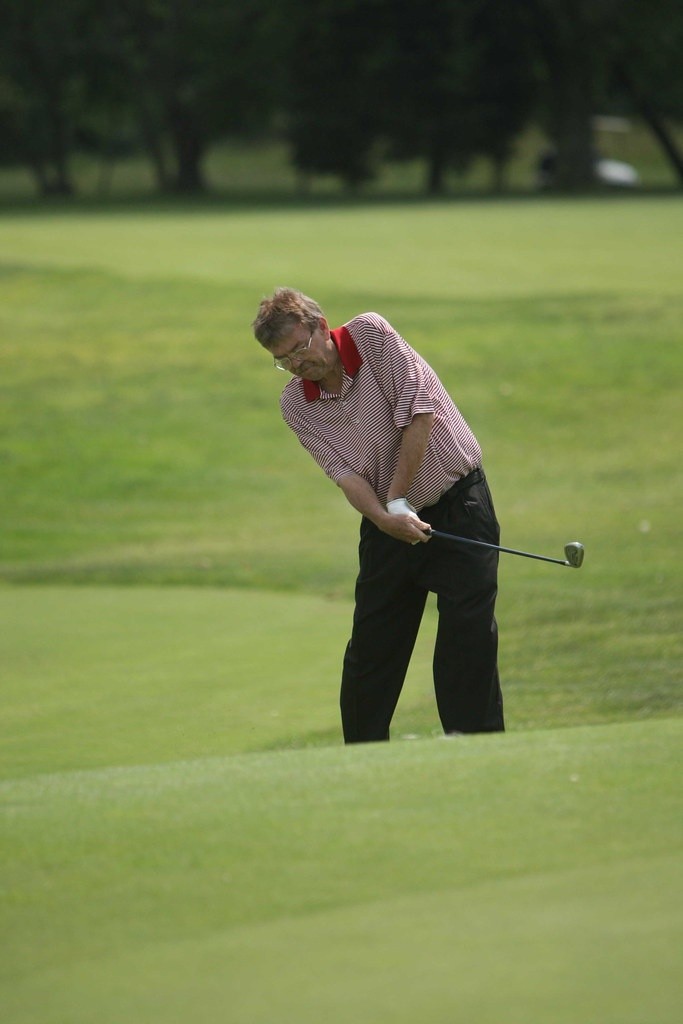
[254,287,507,745]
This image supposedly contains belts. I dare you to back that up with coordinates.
[418,470,487,517]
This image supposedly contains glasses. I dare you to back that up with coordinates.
[273,330,316,371]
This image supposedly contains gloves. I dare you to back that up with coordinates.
[386,497,421,546]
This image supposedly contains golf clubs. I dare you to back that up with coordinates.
[426,527,586,570]
